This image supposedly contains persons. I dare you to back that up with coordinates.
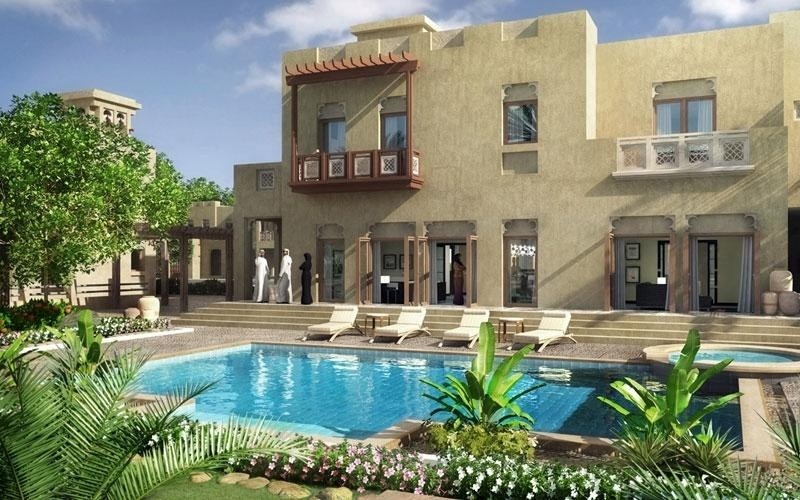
[253,248,313,305]
[453,252,466,304]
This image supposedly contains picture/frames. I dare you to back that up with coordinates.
[624,243,641,284]
[383,253,414,270]
[333,249,343,278]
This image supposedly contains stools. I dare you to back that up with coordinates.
[382,286,397,302]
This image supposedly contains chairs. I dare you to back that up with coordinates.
[636,282,665,309]
[301,304,577,353]
